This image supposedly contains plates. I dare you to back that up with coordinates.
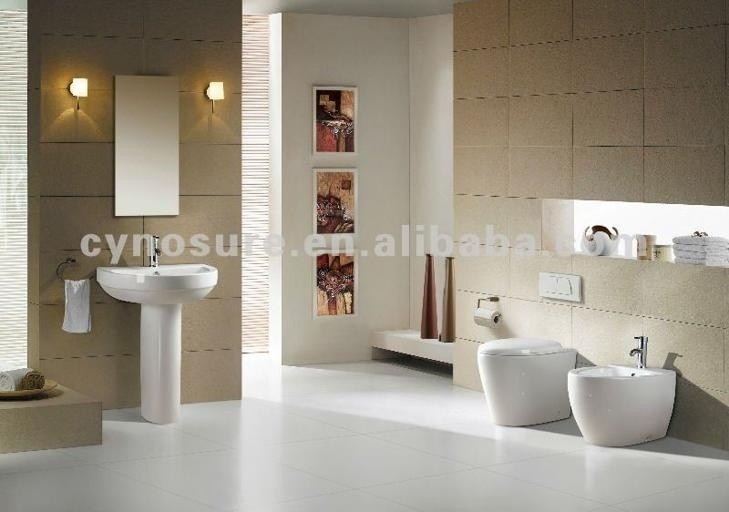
[0,382,58,398]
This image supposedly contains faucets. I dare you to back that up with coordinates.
[627,335,648,368]
[150,235,162,267]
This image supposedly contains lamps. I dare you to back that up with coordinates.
[205,81,224,115]
[69,77,88,111]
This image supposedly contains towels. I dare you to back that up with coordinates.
[0,366,34,391]
[672,235,729,268]
[61,278,93,333]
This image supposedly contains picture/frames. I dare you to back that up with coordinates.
[311,85,359,319]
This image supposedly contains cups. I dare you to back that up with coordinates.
[638,234,673,260]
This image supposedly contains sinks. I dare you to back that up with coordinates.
[96,263,219,305]
[567,365,677,447]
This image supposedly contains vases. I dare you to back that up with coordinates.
[420,253,456,344]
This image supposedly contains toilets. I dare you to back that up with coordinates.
[477,337,579,427]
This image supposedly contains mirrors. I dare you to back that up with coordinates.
[114,75,181,217]
